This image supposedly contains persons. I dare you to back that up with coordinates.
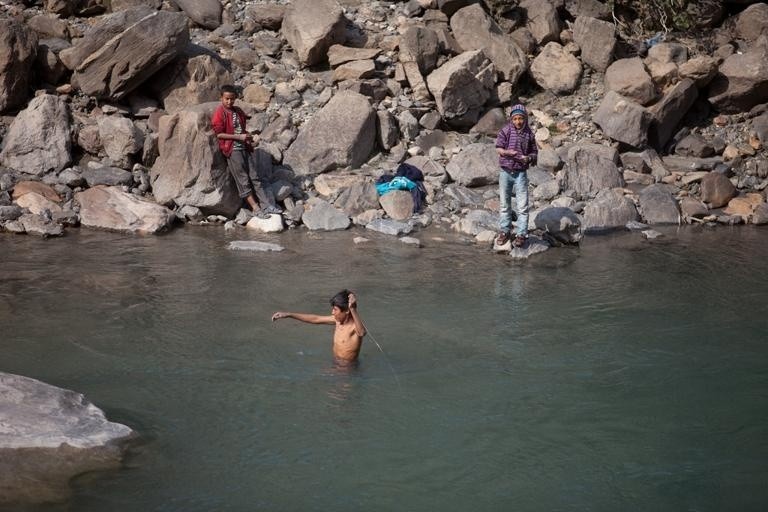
[210,83,284,220]
[312,365,360,428]
[269,289,369,371]
[493,103,537,246]
[491,256,528,368]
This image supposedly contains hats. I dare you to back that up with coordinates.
[511,110,527,117]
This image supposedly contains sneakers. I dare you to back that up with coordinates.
[253,209,272,219]
[265,205,283,215]
[497,232,511,246]
[516,235,525,246]
